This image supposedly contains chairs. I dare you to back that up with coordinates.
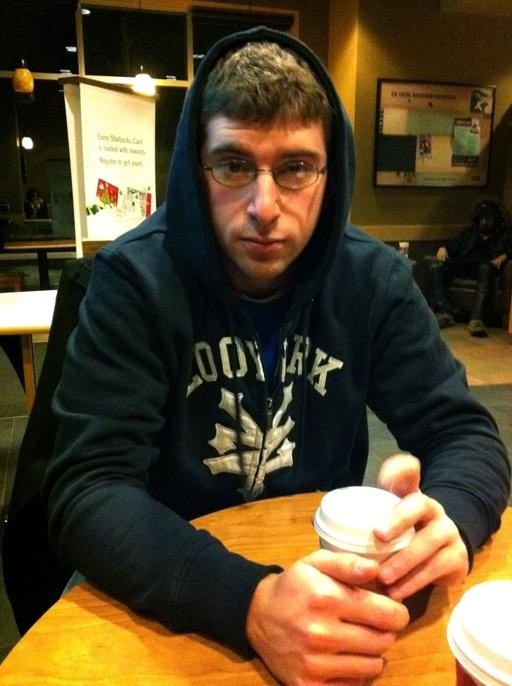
[421,254,512,335]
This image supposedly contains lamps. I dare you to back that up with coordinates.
[133,65,156,96]
[13,59,36,104]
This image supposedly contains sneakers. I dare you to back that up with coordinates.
[434,313,455,328]
[467,319,487,337]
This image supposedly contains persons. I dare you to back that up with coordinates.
[40,24,512,686]
[428,197,512,336]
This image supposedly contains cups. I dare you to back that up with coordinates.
[399,242,409,258]
[446,581,511,686]
[314,486,415,603]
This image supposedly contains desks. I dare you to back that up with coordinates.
[0,491,512,686]
[0,289,58,416]
[0,240,77,289]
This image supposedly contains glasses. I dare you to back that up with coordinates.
[200,154,333,192]
